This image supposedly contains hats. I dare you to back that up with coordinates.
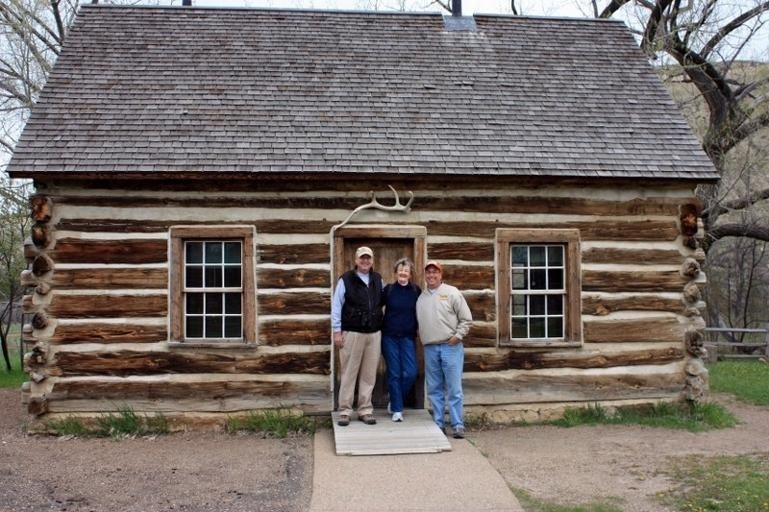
[356,247,373,259]
[425,261,442,271]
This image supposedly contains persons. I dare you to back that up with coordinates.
[331,246,387,426]
[415,260,473,438]
[380,257,421,422]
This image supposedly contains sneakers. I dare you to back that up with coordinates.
[389,400,404,421]
[453,426,465,438]
[359,414,376,424]
[338,415,350,426]
